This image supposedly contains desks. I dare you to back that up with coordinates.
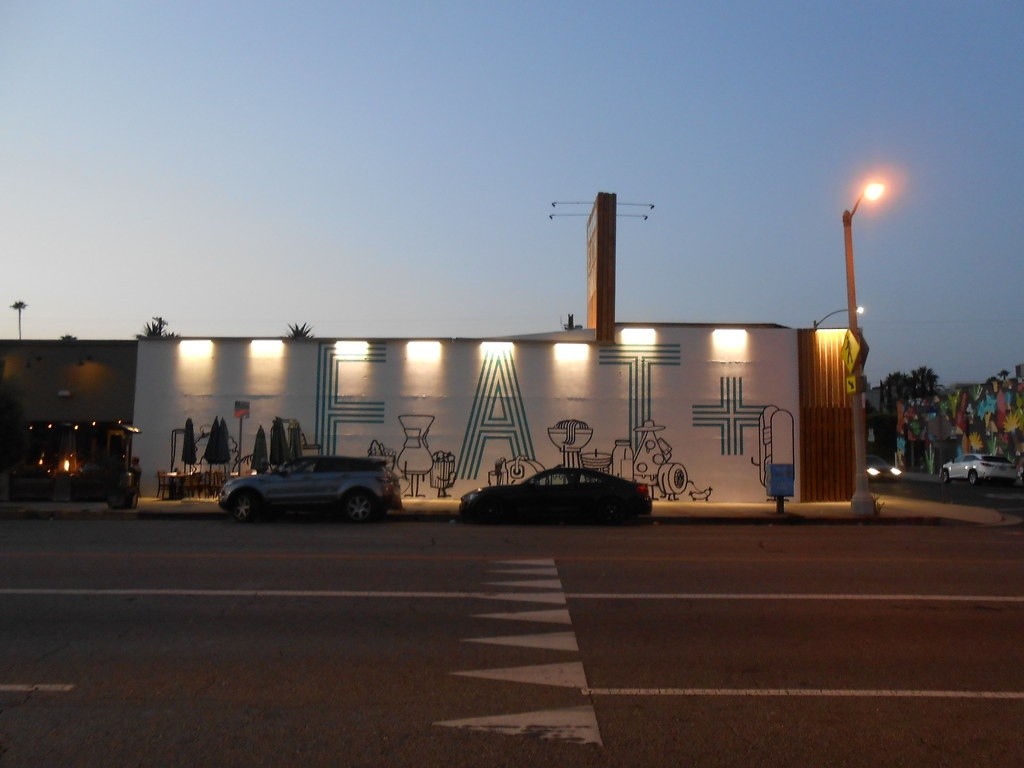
[164,472,188,500]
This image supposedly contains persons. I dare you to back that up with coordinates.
[130,456,142,481]
[85,458,100,472]
[920,455,925,472]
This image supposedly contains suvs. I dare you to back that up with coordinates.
[219,454,395,525]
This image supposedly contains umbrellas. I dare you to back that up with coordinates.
[204,416,231,485]
[269,418,289,465]
[287,421,302,458]
[181,418,197,474]
[251,425,268,474]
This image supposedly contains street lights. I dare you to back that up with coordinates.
[842,183,887,510]
[813,306,864,329]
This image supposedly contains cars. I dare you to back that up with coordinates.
[940,452,1018,487]
[866,454,895,482]
[458,467,652,527]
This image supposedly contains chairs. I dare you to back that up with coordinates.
[158,469,226,499]
